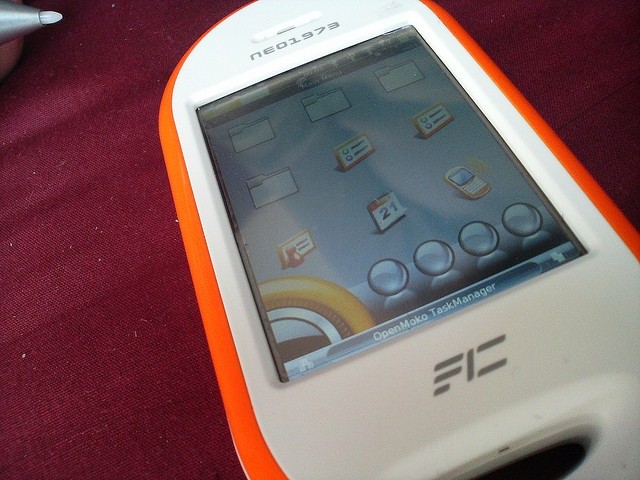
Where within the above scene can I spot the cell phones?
[157,0,640,480]
[445,166,492,201]
